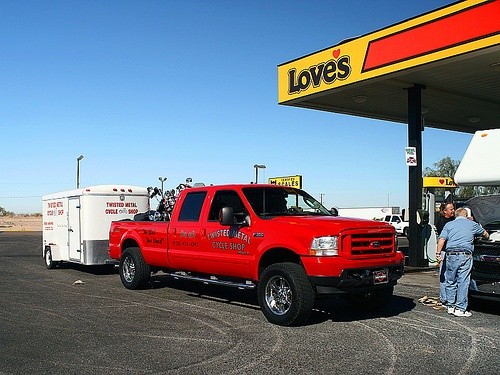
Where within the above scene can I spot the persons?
[433,202,489,317]
[158,200,171,222]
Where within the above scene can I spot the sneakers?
[454,309,472,317]
[447,306,456,315]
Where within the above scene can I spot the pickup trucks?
[373,214,411,236]
[108,182,406,326]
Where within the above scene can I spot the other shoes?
[442,303,448,309]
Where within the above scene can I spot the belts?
[447,252,471,255]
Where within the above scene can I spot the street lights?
[159,177,167,198]
[76,155,84,189]
[253,164,266,184]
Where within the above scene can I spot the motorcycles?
[132,183,193,220]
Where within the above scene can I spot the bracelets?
[436,252,441,256]
